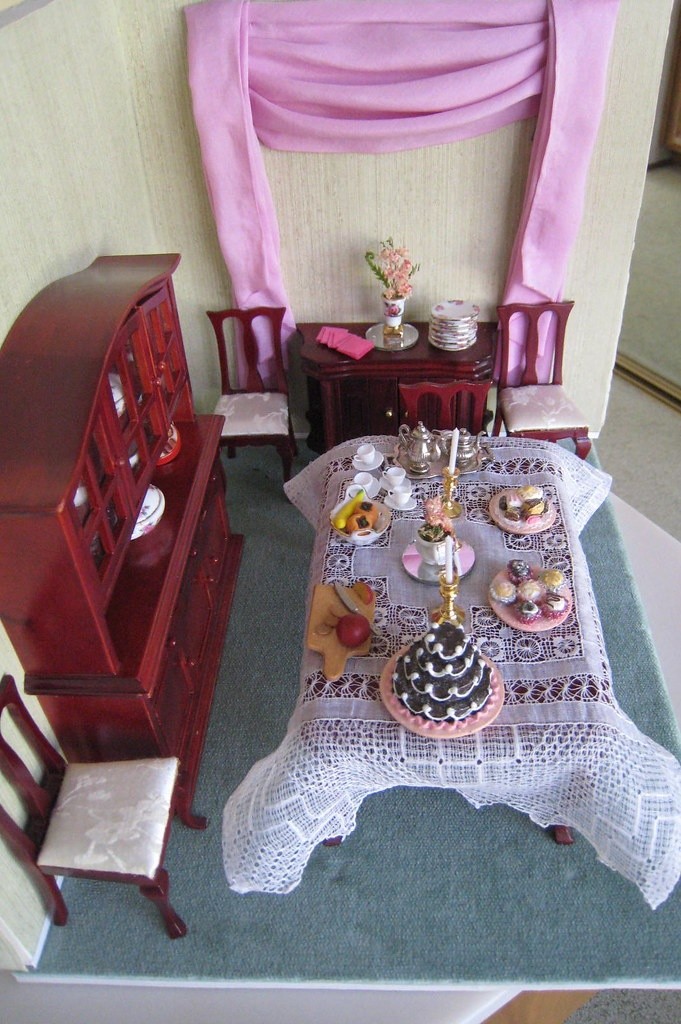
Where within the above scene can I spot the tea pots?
[399,421,441,474]
[441,428,487,468]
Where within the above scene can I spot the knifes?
[334,581,381,635]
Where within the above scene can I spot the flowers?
[364,236,421,300]
[417,496,455,542]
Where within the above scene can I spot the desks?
[221,436,681,912]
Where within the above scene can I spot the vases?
[381,293,406,338]
[415,537,455,566]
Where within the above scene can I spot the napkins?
[316,324,375,360]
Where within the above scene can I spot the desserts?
[498,484,549,522]
[490,560,569,624]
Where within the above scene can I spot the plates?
[379,477,411,491]
[383,495,417,511]
[349,478,380,499]
[489,490,556,534]
[352,451,383,471]
[428,300,480,351]
[379,646,505,739]
[487,567,573,632]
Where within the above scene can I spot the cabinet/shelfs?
[0,253,246,829]
[296,320,504,454]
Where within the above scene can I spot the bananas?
[333,489,364,527]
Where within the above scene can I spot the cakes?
[392,622,493,721]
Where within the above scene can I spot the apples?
[353,582,373,603]
[336,613,370,647]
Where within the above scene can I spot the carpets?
[12,435,681,985]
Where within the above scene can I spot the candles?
[445,535,454,585]
[448,427,459,475]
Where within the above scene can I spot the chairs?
[492,300,591,463]
[0,674,208,939]
[204,306,293,483]
[397,382,493,434]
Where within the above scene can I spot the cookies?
[316,608,347,635]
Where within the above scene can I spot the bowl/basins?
[131,483,165,540]
[329,484,391,546]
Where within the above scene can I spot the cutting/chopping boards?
[307,584,376,681]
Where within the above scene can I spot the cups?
[388,485,412,506]
[353,472,373,492]
[354,444,376,465]
[382,466,406,486]
[414,521,445,566]
